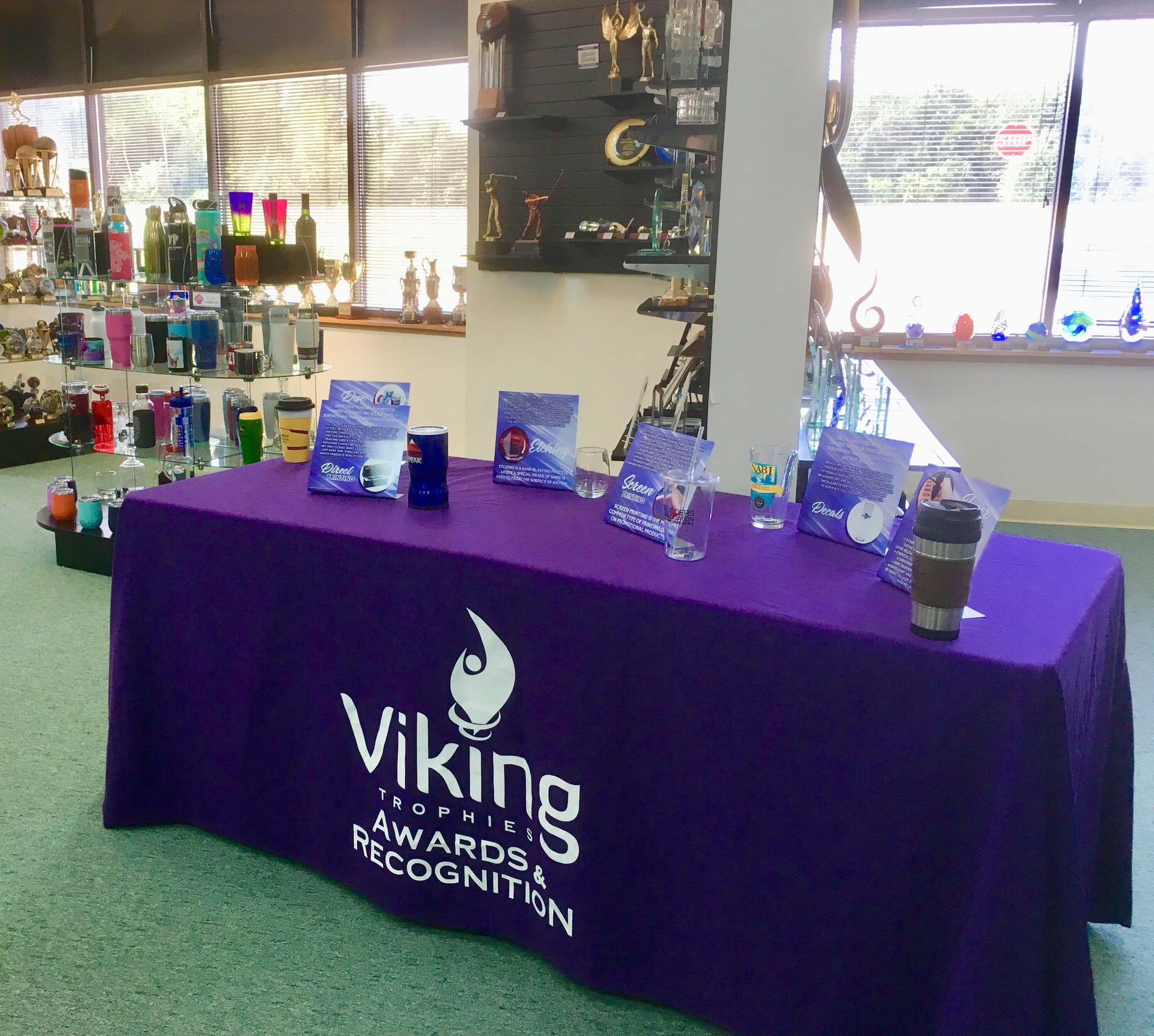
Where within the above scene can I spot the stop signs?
[994,122,1035,161]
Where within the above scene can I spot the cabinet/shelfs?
[35,266,332,578]
[462,0,722,271]
[612,0,732,464]
[0,193,101,468]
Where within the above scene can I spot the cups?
[660,469,721,560]
[130,334,155,367]
[133,410,156,449]
[234,349,273,376]
[909,500,982,640]
[205,248,229,284]
[166,337,203,372]
[573,447,609,498]
[79,338,105,367]
[407,425,450,510]
[46,472,123,532]
[228,342,254,371]
[275,397,312,463]
[111,402,134,431]
[750,445,798,529]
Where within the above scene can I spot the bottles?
[51,169,326,465]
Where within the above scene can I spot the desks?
[125,451,1134,1036]
[795,360,961,505]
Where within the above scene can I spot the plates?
[0,277,66,422]
